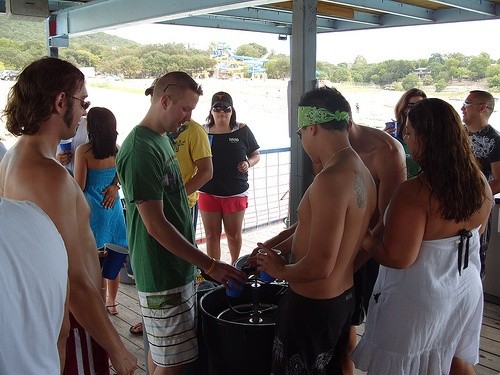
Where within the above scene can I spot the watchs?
[111,181,121,189]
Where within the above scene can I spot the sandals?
[129,322,143,336]
[106,305,119,315]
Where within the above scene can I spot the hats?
[210,91,233,108]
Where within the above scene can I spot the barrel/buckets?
[197,282,284,375]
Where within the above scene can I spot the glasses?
[212,105,231,112]
[163,83,202,93]
[462,101,481,108]
[296,126,306,140]
[72,96,91,110]
[406,103,416,108]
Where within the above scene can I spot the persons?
[348,119,406,318]
[197,92,259,266]
[115,71,243,375]
[384,87,426,181]
[0,56,138,375]
[353,98,494,375]
[245,87,377,375]
[461,90,500,283]
[144,75,213,247]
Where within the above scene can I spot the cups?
[102,243,129,280]
[208,135,213,146]
[60,142,71,152]
[385,122,397,138]
[258,249,281,282]
[226,277,243,298]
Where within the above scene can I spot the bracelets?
[245,159,250,167]
[204,258,216,275]
